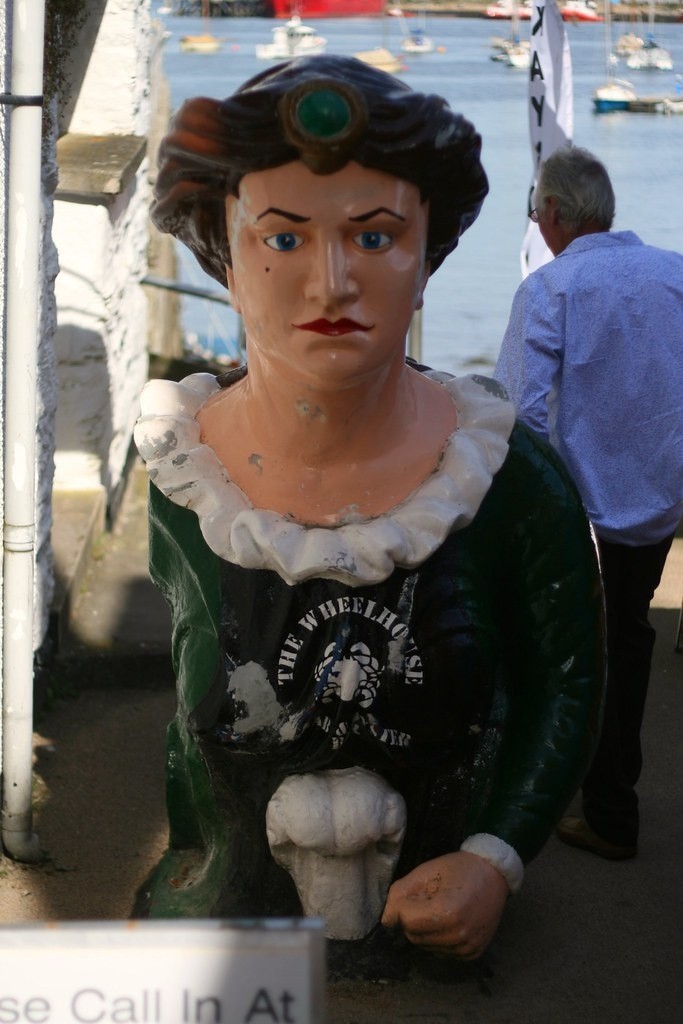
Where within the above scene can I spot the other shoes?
[557,816,639,861]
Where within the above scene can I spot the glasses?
[527,205,543,223]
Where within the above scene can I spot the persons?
[35,54,604,953]
[504,146,683,860]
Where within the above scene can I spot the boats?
[402,29,433,53]
[483,0,532,20]
[559,1,603,22]
[255,15,327,61]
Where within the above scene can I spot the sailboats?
[490,0,532,68]
[592,0,683,115]
